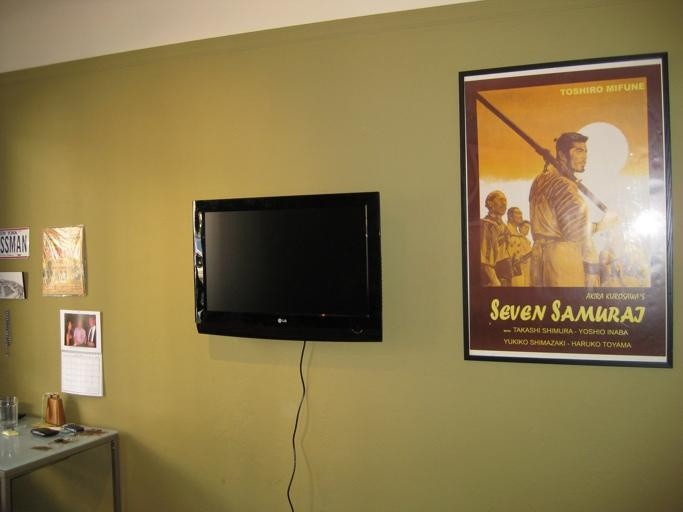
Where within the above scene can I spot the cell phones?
[63,424,83,433]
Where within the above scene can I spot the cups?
[0,397,19,428]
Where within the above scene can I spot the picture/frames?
[457,52,674,368]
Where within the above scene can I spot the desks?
[1,417,121,510]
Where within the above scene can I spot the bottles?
[41,393,66,427]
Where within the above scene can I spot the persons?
[66,317,96,347]
[480,133,620,288]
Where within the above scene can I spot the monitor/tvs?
[193,191,382,342]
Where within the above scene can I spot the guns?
[477,94,609,213]
